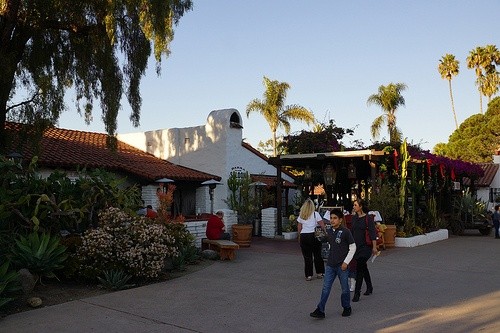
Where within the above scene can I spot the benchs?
[201,238,239,260]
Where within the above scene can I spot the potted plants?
[367,182,398,248]
[224,171,264,247]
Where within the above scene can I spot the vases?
[282,232,298,240]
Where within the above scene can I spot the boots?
[349,277,356,291]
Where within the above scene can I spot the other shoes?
[342,307,352,317]
[364,287,373,295]
[310,308,325,318]
[352,293,360,302]
[306,275,313,280]
[316,274,325,278]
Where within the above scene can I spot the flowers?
[282,215,296,232]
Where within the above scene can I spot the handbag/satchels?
[313,211,328,242]
[365,214,381,246]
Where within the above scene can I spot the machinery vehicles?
[408,179,495,237]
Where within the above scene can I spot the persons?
[135,200,158,220]
[351,199,378,302]
[206,211,231,241]
[309,208,357,319]
[296,198,327,281]
[491,205,500,239]
